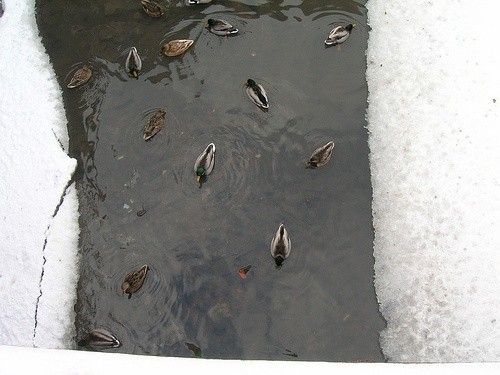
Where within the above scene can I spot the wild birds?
[189,0,212,4]
[142,110,167,140]
[203,18,239,36]
[125,47,142,78]
[270,224,292,267]
[160,39,194,57]
[82,329,120,348]
[243,78,270,108]
[67,64,93,89]
[307,141,334,168]
[193,143,216,182]
[120,264,148,294]
[139,0,165,17]
[324,23,356,45]
